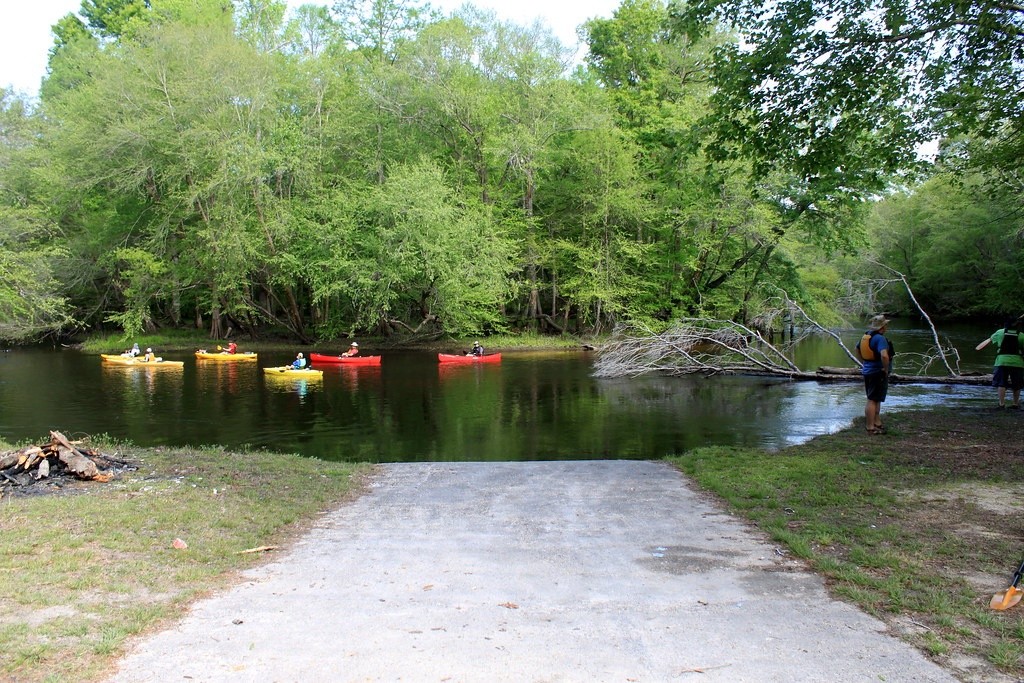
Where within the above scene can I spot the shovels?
[989,557,1024,611]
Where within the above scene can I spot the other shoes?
[1012,405,1020,408]
[998,403,1006,410]
[874,422,885,430]
[864,427,887,435]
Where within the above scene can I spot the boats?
[194,350,258,361]
[263,366,324,376]
[438,352,502,362]
[101,352,185,367]
[309,352,381,364]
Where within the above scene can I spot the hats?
[228,339,234,343]
[147,348,152,352]
[351,341,358,346]
[473,341,479,344]
[867,315,891,332]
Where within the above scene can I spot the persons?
[140,348,154,362]
[990,315,1024,411]
[473,341,484,354]
[286,352,306,370]
[221,340,237,354]
[121,343,140,358]
[341,341,359,357]
[856,315,895,435]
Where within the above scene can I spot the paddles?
[990,558,1024,611]
[974,312,1024,350]
[216,344,225,352]
